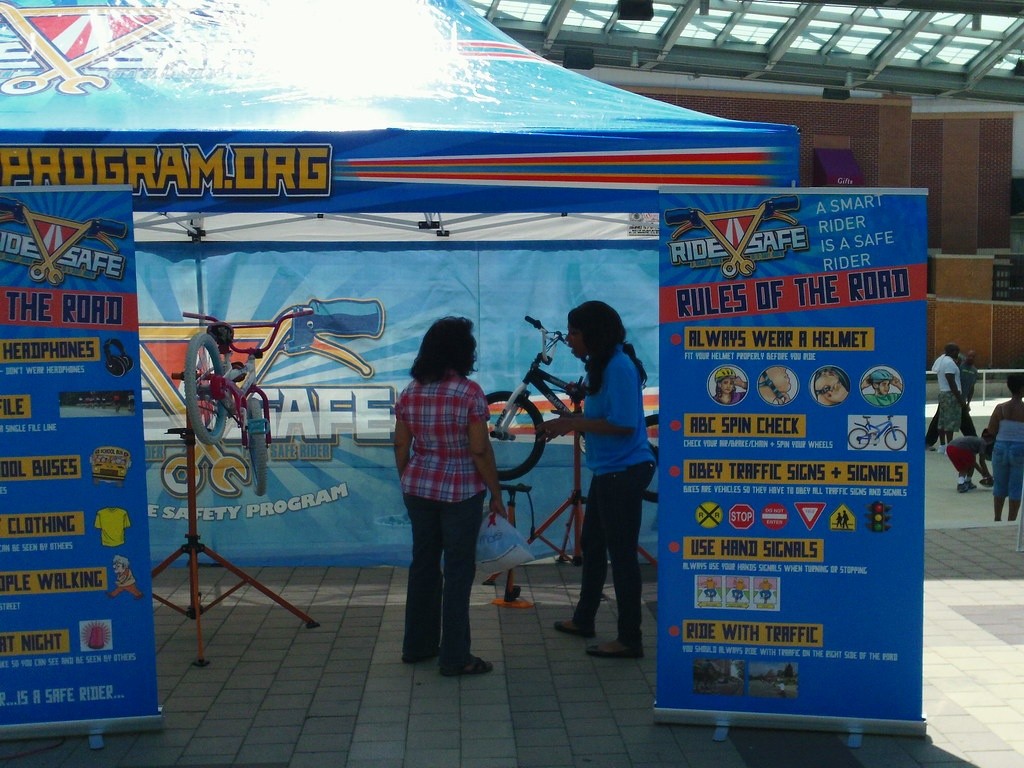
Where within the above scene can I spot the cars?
[848,414,908,451]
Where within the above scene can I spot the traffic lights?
[864,501,892,534]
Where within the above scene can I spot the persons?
[535,301,656,657]
[777,681,784,693]
[936,343,963,455]
[947,428,993,492]
[925,350,978,451]
[988,373,1024,521]
[393,317,507,675]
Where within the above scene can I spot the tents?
[0,0,800,567]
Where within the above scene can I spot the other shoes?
[963,480,977,489]
[957,483,969,492]
[402,646,441,663]
[586,645,643,658]
[555,622,595,636]
[937,445,946,454]
[925,444,935,451]
[440,657,492,676]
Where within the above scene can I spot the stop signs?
[761,505,790,530]
[729,504,755,530]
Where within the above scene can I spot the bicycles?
[174,302,317,496]
[471,314,663,504]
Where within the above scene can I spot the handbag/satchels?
[470,510,536,586]
